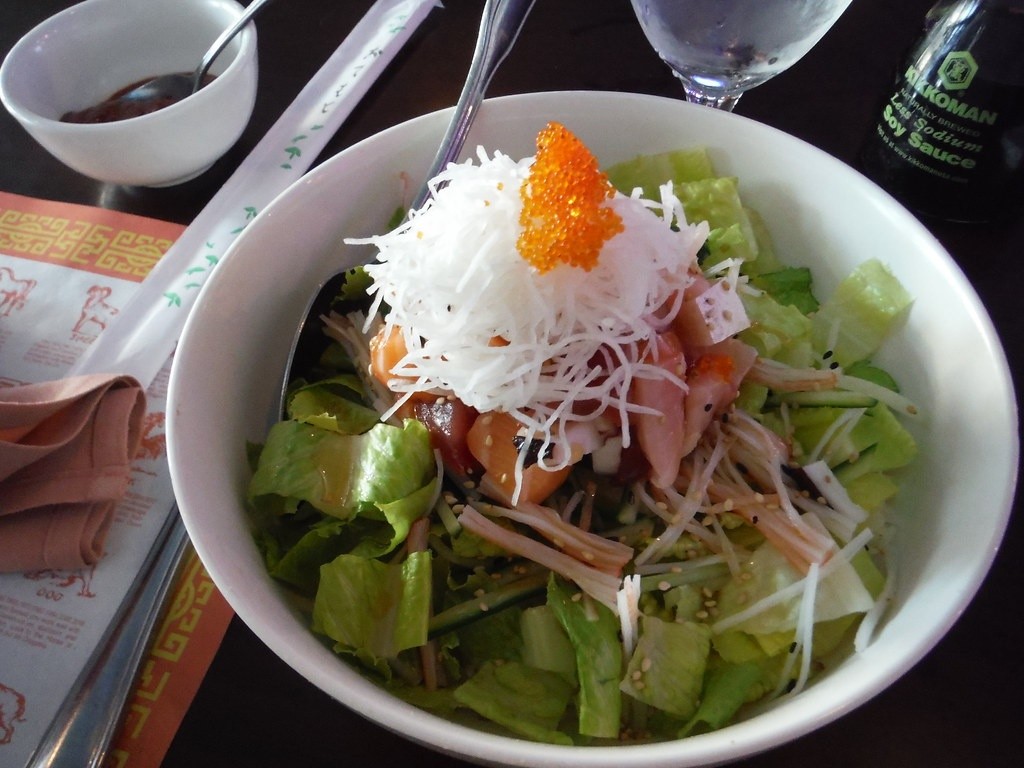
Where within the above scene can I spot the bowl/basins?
[0,0,258,188]
[166,90,1017,768]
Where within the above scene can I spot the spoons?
[279,2,538,425]
[118,0,270,98]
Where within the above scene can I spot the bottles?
[846,0,1024,224]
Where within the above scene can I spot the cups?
[628,0,855,112]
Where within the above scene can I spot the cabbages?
[239,146,915,745]
